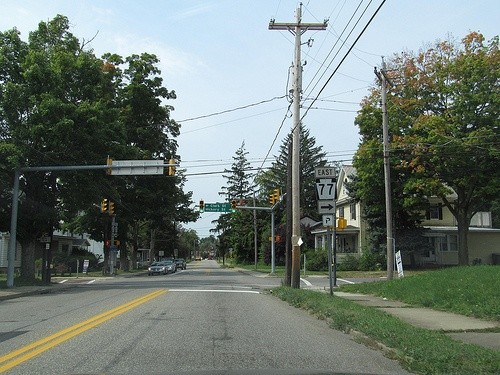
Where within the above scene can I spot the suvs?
[175,258,186,270]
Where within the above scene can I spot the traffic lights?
[101,199,108,213]
[231,200,237,213]
[109,201,114,216]
[199,201,204,213]
[106,240,110,246]
[274,188,280,201]
[270,194,275,205]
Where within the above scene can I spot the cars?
[148,261,176,275]
